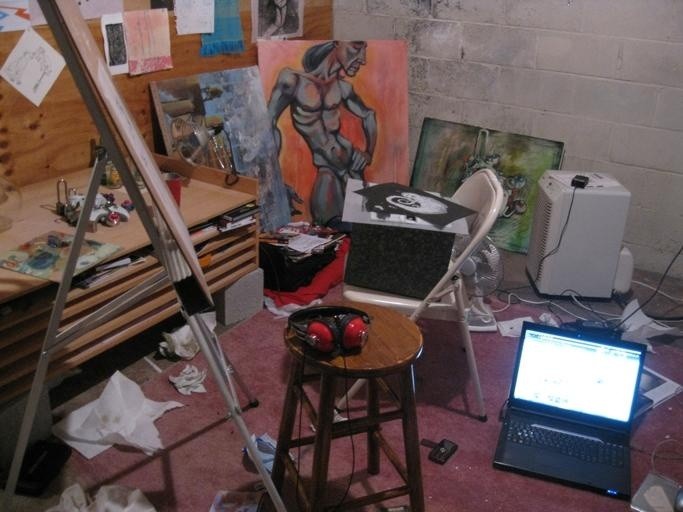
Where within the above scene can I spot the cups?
[162,171,180,209]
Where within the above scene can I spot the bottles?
[93,138,145,188]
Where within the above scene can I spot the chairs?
[330,167,506,423]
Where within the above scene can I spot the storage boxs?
[339,177,453,301]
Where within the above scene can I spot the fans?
[458,233,503,331]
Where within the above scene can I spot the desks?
[0,146,263,458]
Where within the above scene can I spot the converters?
[571,175,589,188]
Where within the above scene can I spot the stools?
[271,300,426,511]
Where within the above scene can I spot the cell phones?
[429,439,457,465]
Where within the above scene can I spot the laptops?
[493,321,647,500]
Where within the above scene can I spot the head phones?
[288,306,369,360]
